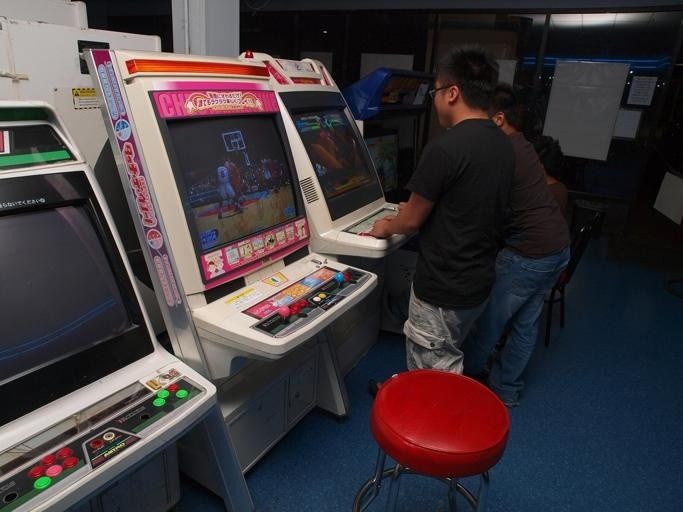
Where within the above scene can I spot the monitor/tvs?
[0,171,154,428]
[278,91,383,222]
[147,89,311,285]
[363,113,419,193]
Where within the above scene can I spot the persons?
[217,161,243,219]
[369,46,513,400]
[465,83,572,406]
[529,134,567,221]
[224,157,242,207]
[267,154,282,194]
[319,114,335,136]
[261,156,273,195]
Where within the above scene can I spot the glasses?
[429,83,461,98]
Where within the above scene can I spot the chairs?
[540,224,589,349]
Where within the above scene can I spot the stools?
[351,368,510,511]
